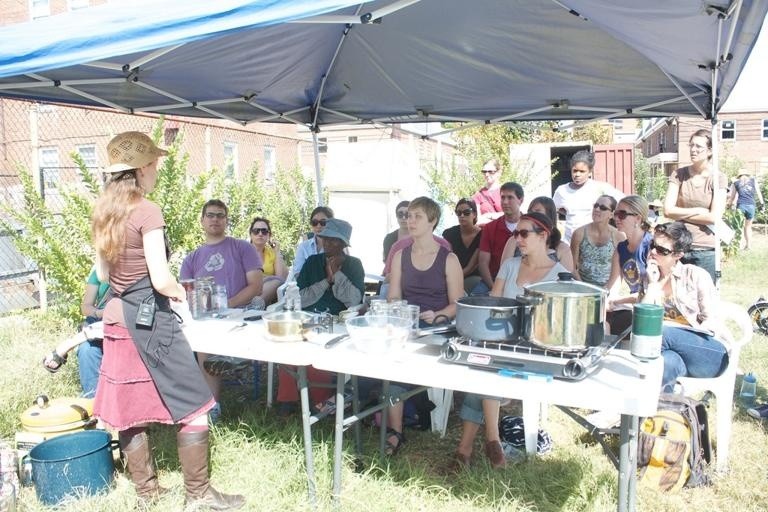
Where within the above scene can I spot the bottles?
[631,302,663,359]
[284,279,302,314]
[739,370,757,406]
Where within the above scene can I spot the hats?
[316,218,352,248]
[103,130,170,176]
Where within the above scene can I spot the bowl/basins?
[344,317,412,354]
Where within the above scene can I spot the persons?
[725,167,766,250]
[90,131,246,511]
[42,270,110,399]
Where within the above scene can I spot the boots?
[118,425,176,510]
[176,428,245,512]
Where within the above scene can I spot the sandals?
[313,393,352,417]
[385,426,405,457]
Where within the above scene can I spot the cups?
[370,297,421,331]
[179,277,230,319]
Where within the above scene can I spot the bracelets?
[93,308,98,321]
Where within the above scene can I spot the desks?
[169,300,362,502]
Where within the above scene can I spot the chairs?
[216,296,265,399]
[266,282,366,411]
[378,283,467,436]
[638,300,752,473]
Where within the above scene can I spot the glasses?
[251,228,269,236]
[310,218,327,227]
[613,209,638,220]
[481,170,499,175]
[649,240,676,257]
[204,211,226,219]
[512,228,543,239]
[396,211,408,219]
[594,203,611,212]
[454,207,473,217]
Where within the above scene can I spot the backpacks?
[636,379,714,491]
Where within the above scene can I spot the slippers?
[42,345,71,373]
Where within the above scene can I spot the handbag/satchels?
[495,412,553,459]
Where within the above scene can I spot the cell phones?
[244,315,262,321]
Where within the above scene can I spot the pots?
[452,294,524,341]
[17,395,119,509]
[519,270,609,353]
[262,311,306,343]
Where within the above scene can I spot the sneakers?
[209,403,221,423]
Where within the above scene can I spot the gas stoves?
[444,335,611,379]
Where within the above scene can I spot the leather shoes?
[436,450,471,477]
[485,439,508,472]
